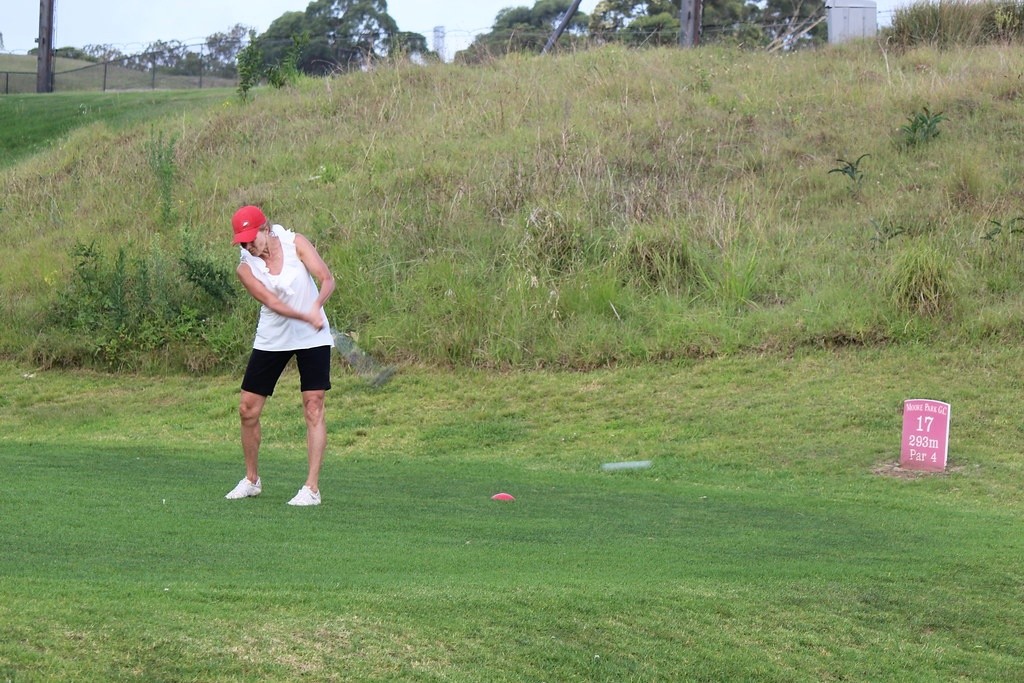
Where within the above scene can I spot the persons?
[226,206,336,506]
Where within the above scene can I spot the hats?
[232,206,266,246]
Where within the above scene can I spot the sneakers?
[225,476,262,499]
[287,485,321,506]
